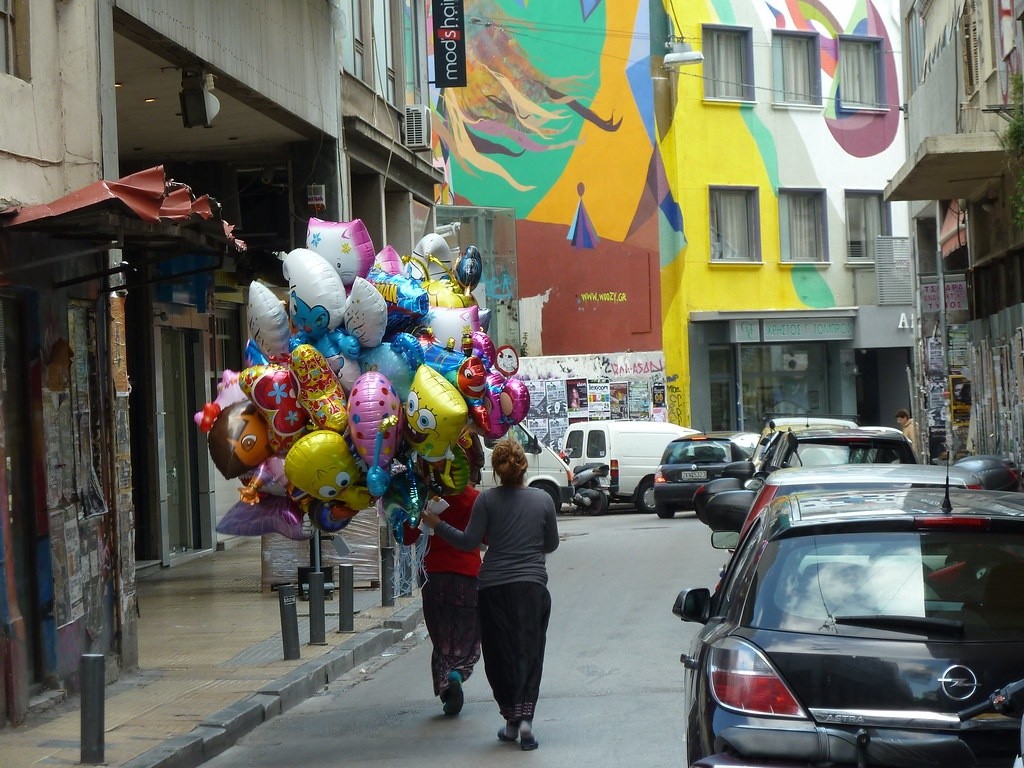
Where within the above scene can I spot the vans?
[563,419,730,513]
[470,418,576,514]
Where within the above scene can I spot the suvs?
[669,488,1024,768]
[691,414,986,597]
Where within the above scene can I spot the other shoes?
[443,670,464,715]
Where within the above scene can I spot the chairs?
[801,562,873,603]
[979,564,1024,613]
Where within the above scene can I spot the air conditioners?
[404,104,432,150]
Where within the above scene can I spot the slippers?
[521,736,538,750]
[498,728,514,742]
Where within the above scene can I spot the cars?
[654,429,762,519]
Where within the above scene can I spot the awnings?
[0,161,249,293]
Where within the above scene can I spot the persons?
[895,409,920,462]
[421,438,559,750]
[569,387,581,407]
[392,483,487,716]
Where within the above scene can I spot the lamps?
[663,36,704,67]
[179,69,221,127]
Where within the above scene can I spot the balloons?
[193,218,530,547]
